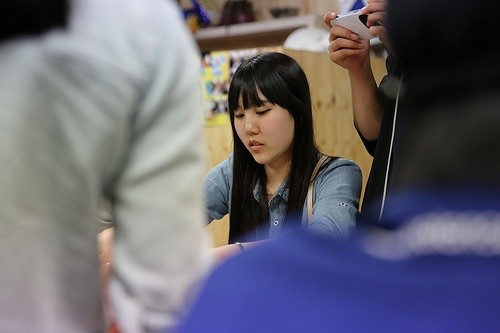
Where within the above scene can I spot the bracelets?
[234,241,248,255]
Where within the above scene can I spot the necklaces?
[264,187,280,197]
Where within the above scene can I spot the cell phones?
[330,7,384,40]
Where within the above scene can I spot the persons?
[322,0,403,232]
[169,0,500,332]
[0,0,209,333]
[96,50,363,313]
